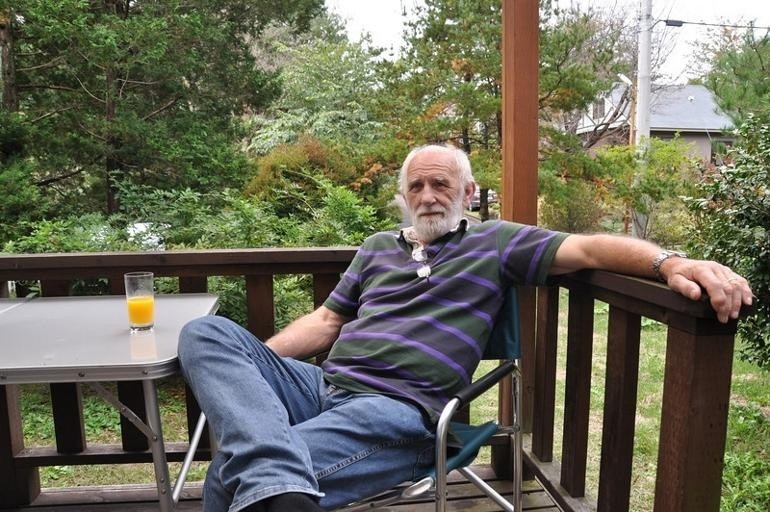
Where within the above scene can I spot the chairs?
[344,283,533,511]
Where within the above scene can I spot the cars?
[468,185,497,211]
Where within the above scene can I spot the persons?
[177,143,753,512]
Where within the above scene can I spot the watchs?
[651,249,688,285]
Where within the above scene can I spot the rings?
[726,277,737,282]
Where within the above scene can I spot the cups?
[124,271,155,332]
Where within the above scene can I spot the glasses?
[411,246,433,286]
